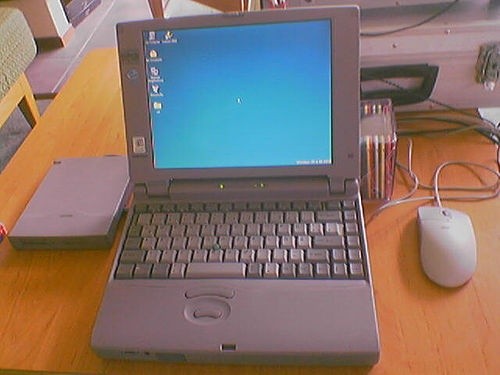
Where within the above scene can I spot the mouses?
[417,205,477,288]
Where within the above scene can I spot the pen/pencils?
[360,103,392,201]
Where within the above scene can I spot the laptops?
[90,4,380,365]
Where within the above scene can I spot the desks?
[1,45,500,375]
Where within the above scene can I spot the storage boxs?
[360,2,500,113]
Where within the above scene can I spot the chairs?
[2,5,42,137]
